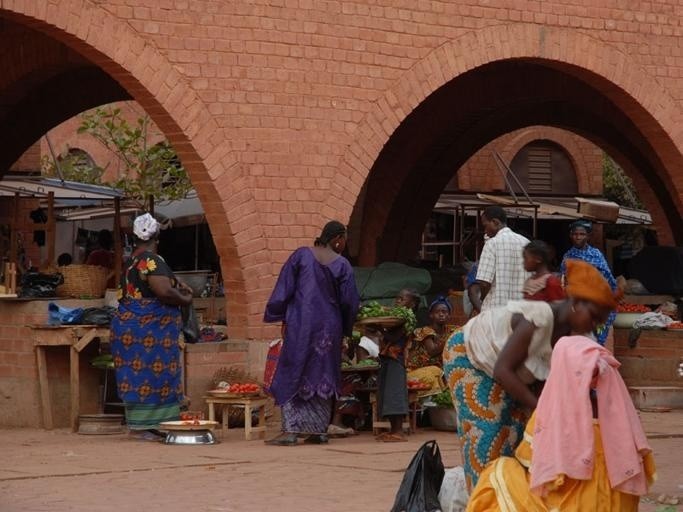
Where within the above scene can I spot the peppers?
[355,301,390,320]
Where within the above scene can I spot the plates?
[204,388,260,399]
[159,420,222,430]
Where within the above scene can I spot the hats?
[569,218,591,231]
[564,257,617,308]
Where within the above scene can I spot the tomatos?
[228,382,263,396]
[408,380,432,390]
[615,302,652,312]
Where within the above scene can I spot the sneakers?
[306,434,330,444]
[327,423,355,436]
[264,433,296,447]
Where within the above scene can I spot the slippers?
[131,429,164,443]
[373,429,406,443]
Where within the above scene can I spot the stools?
[203,395,269,442]
[369,392,417,436]
[0,262,18,298]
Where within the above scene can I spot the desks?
[24,324,111,433]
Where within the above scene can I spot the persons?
[522,240,567,301]
[462,334,657,512]
[442,258,617,499]
[560,221,616,349]
[475,206,536,313]
[58,229,125,298]
[110,212,193,441]
[468,260,490,318]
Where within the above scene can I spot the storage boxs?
[575,196,619,224]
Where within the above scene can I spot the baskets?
[211,366,268,427]
[49,262,109,299]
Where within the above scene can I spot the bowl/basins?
[612,313,651,329]
[163,432,220,446]
[171,269,214,299]
